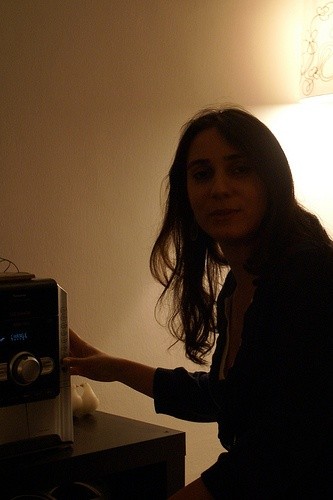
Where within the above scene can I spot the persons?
[58,105,332,500]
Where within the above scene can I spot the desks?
[2,407,188,499]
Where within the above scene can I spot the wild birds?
[71,382,99,419]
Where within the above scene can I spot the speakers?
[0,278,75,462]
